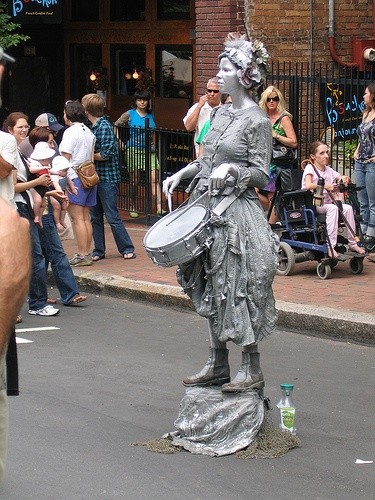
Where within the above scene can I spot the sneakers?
[69,253,93,266]
[28,305,60,316]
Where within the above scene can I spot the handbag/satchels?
[76,160,100,189]
[271,115,297,163]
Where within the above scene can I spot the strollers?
[266,168,370,279]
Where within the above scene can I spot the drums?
[143,204,215,268]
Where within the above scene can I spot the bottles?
[277,384,297,437]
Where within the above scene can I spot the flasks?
[315,177,324,206]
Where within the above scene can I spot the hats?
[35,113,64,131]
[50,156,73,171]
[29,141,56,160]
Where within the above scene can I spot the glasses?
[65,100,74,105]
[11,125,30,129]
[207,89,219,93]
[267,96,279,102]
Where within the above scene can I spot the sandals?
[46,299,57,303]
[92,254,101,260]
[124,253,136,259]
[69,294,87,305]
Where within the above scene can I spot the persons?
[182,78,233,159]
[113,90,167,217]
[301,141,365,257]
[0,93,135,321]
[162,33,280,393]
[0,196,33,498]
[353,84,375,252]
[259,85,297,229]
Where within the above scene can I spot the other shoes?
[129,210,139,217]
[157,209,166,215]
[357,234,375,252]
